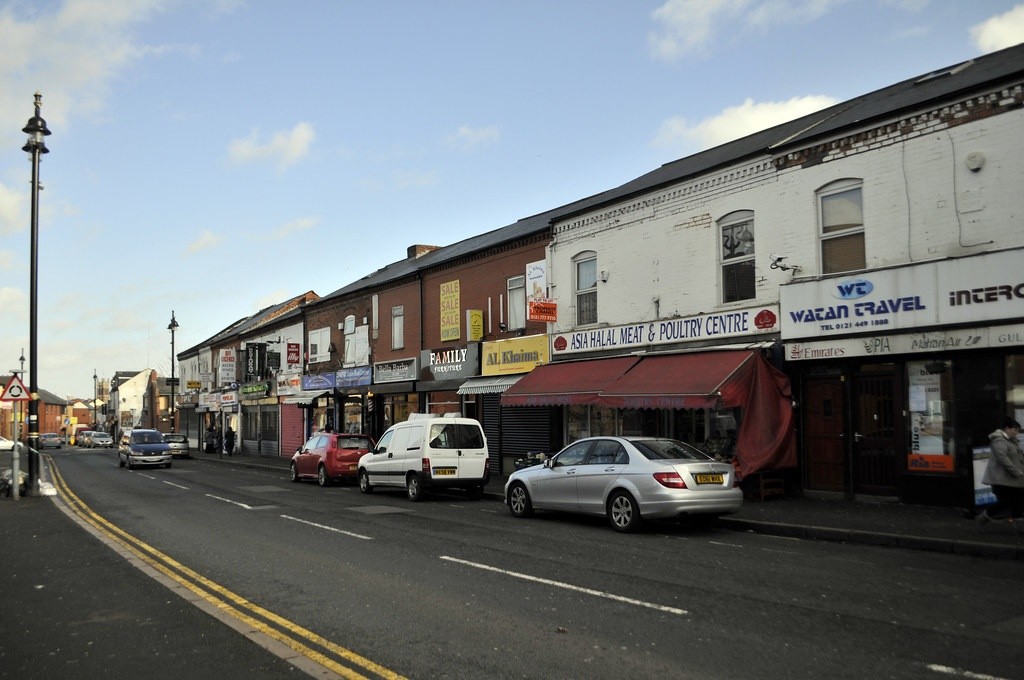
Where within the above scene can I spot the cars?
[503,434,746,535]
[161,433,190,458]
[117,426,173,470]
[0,435,24,453]
[38,428,114,449]
[288,432,378,487]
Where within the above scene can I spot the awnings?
[501,349,761,410]
[456,374,529,395]
[283,390,330,404]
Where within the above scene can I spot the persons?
[117,429,124,439]
[975,416,1024,526]
[205,426,235,457]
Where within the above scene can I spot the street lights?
[18,346,27,443]
[92,368,99,431]
[17,91,53,496]
[166,310,180,434]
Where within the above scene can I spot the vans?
[357,411,491,503]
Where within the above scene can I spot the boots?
[974,511,993,535]
